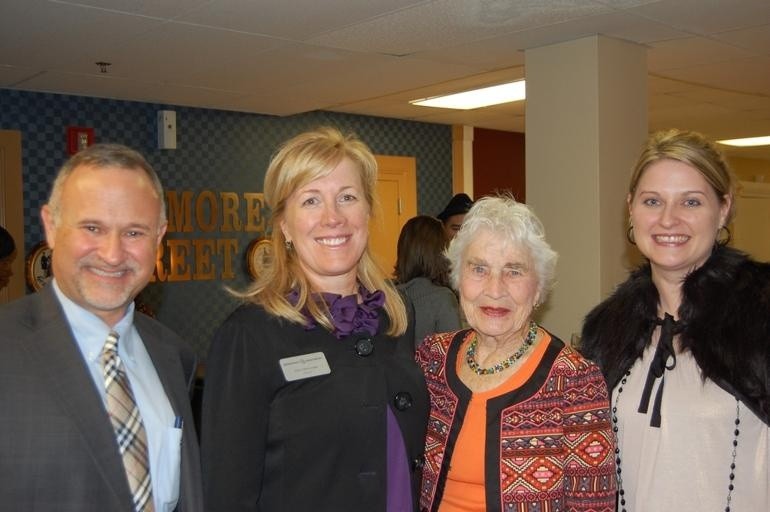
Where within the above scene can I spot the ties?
[102,331,156,511]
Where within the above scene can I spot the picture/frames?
[25,245,51,292]
[245,238,272,281]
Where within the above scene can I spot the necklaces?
[466,320,537,375]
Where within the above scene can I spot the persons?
[199,125,431,511]
[390,215,461,348]
[0,225,17,289]
[436,192,474,241]
[415,195,616,511]
[41,252,47,276]
[579,127,770,512]
[0,143,205,512]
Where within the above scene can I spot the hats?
[436,193,474,219]
